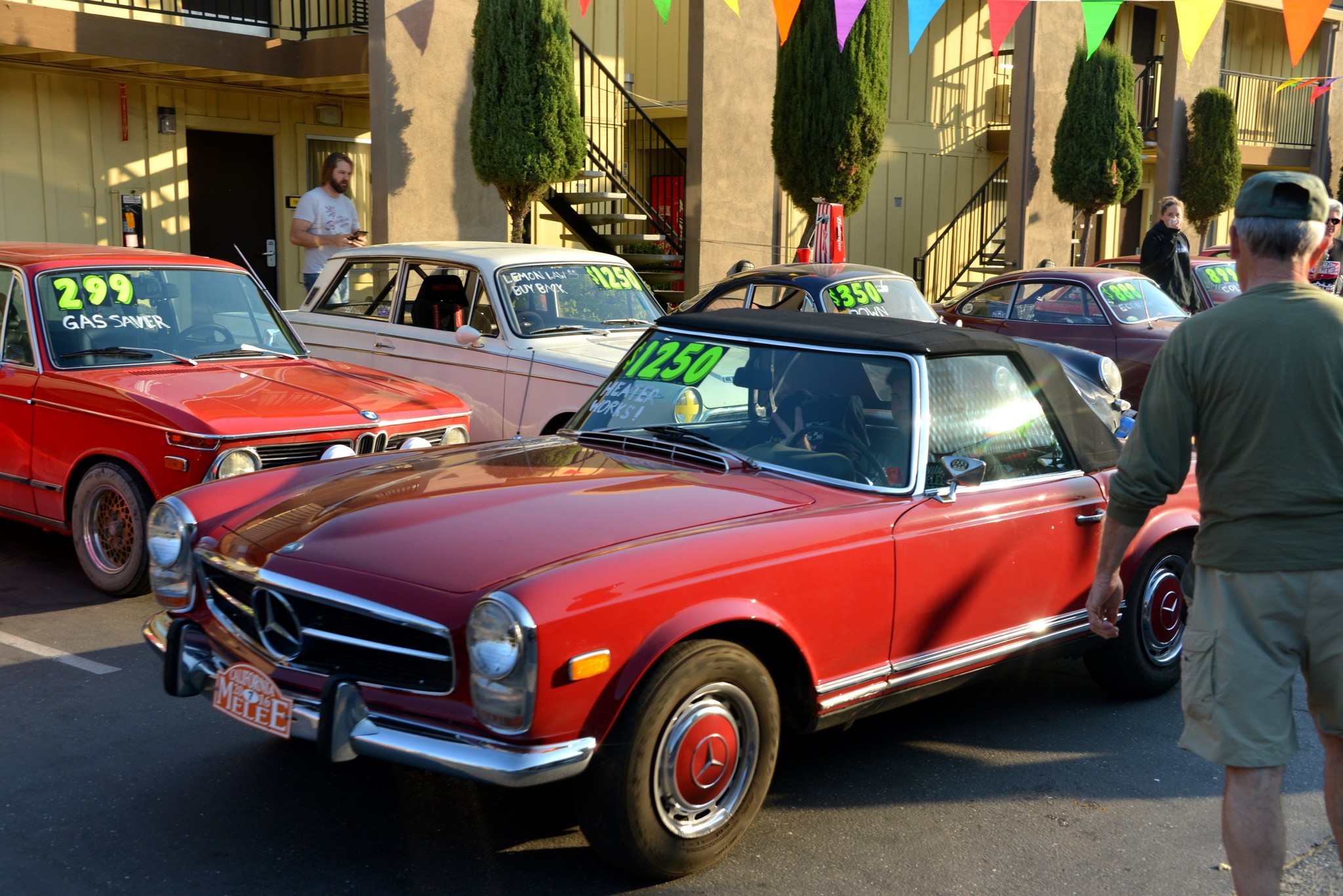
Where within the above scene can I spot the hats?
[1234,170,1330,222]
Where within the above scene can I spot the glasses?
[1326,218,1341,225]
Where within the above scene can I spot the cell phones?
[350,230,368,242]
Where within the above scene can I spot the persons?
[289,152,368,311]
[1086,173,1343,896]
[1141,195,1343,312]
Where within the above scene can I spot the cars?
[2,242,472,596]
[213,242,765,446]
[990,255,1245,314]
[1198,244,1232,259]
[142,308,1204,884]
[911,265,1198,418]
[658,263,1123,438]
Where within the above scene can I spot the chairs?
[411,273,493,335]
[759,383,874,463]
[0,298,181,365]
[962,300,1100,326]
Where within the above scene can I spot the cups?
[797,248,810,262]
